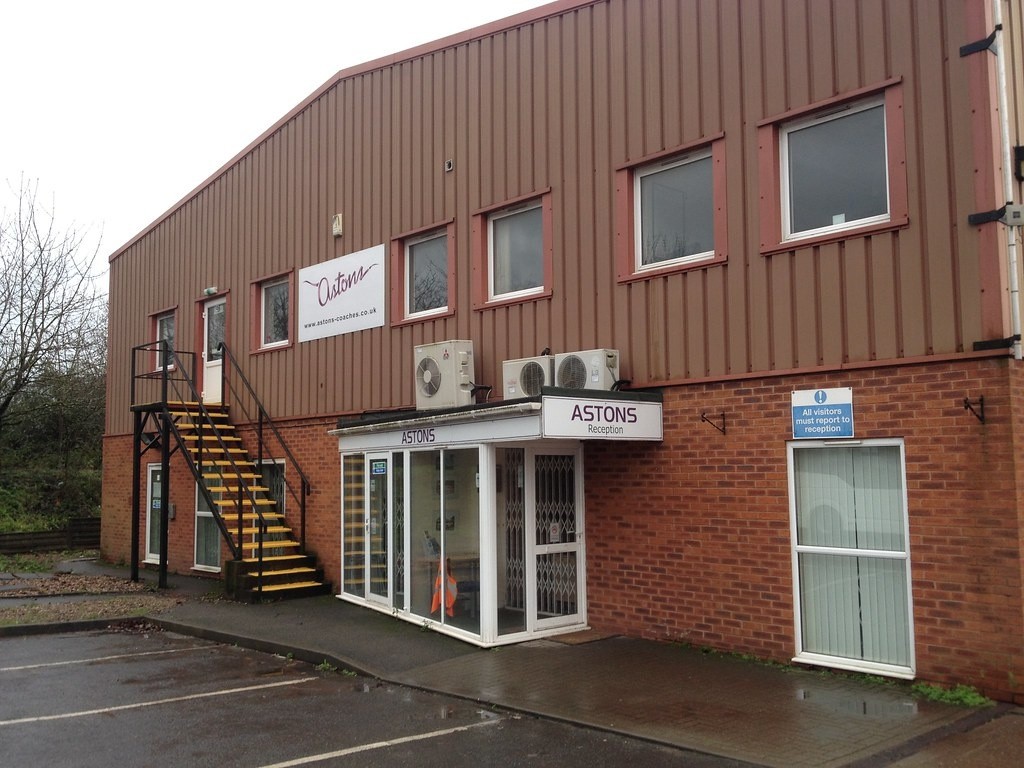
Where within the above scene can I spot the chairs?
[447,557,479,623]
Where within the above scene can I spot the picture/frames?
[431,452,458,474]
[431,475,459,500]
[432,510,460,536]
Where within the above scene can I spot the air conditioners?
[554,349,620,392]
[502,355,555,400]
[412,339,475,412]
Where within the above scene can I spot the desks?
[419,552,480,617]
